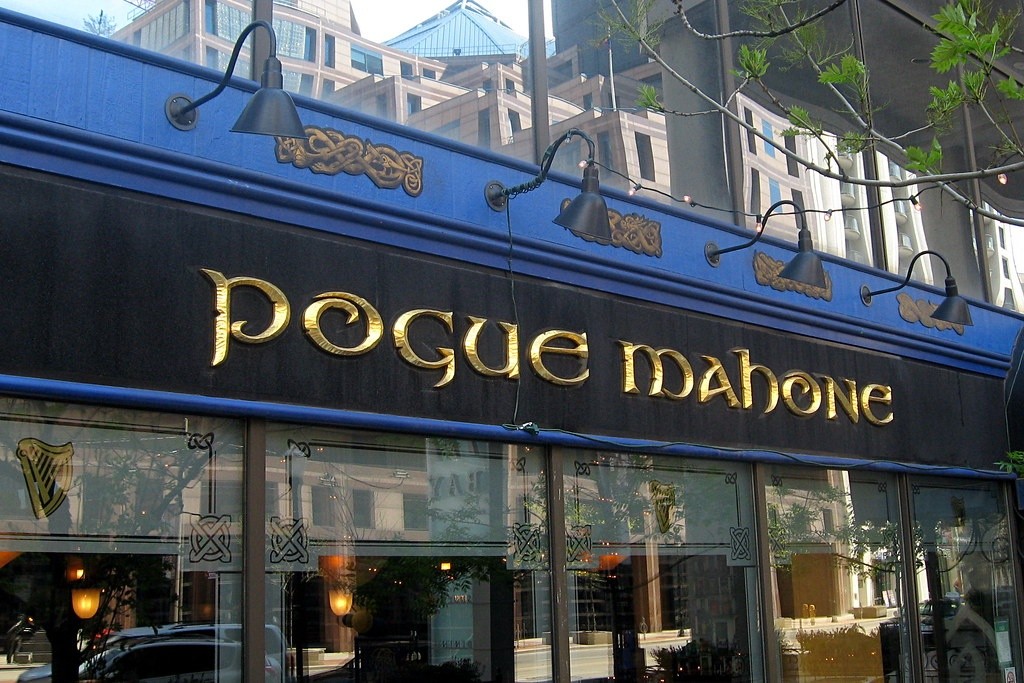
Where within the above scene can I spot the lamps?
[860,250,974,326]
[452,49,461,57]
[484,127,614,242]
[704,200,826,290]
[165,19,307,140]
[328,588,353,616]
[71,588,100,620]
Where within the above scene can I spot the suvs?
[78,637,279,683]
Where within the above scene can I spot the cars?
[875,591,970,634]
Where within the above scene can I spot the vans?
[15,619,295,683]
